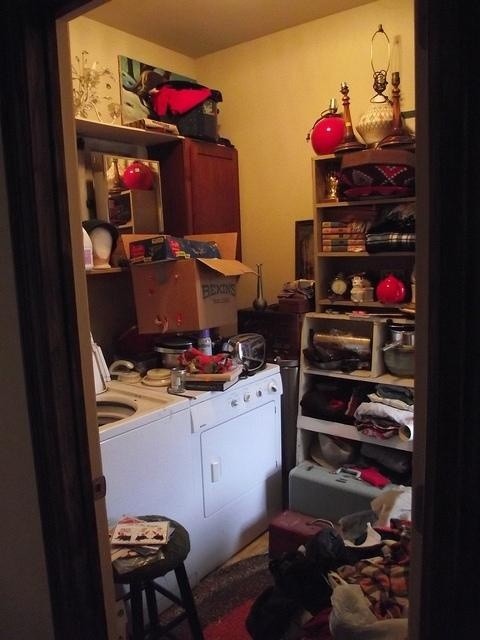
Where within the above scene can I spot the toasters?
[221,332,266,379]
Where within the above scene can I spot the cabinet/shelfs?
[74,117,181,275]
[107,189,159,234]
[148,137,243,281]
[310,142,417,312]
[294,310,415,467]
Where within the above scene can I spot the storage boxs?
[121,231,259,335]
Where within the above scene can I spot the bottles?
[199,330,213,356]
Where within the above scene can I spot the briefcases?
[268,509,338,563]
[288,460,397,526]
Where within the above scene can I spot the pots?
[383,325,415,351]
[153,334,198,369]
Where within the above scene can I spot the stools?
[108,515,203,640]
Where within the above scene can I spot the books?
[109,515,175,561]
[183,366,243,391]
[322,221,366,252]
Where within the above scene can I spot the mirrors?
[78,136,164,235]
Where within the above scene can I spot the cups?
[170,368,186,392]
